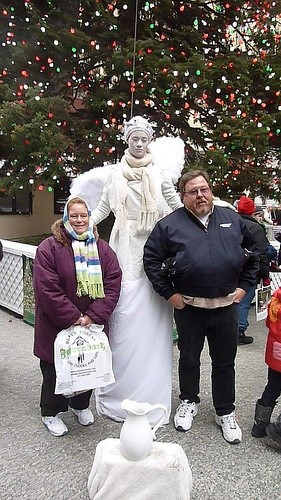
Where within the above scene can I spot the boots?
[251,399,275,438]
[265,413,281,442]
[237,329,254,345]
[270,260,281,272]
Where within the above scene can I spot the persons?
[232,195,281,345]
[143,171,260,444]
[66,115,186,427]
[34,198,123,437]
[250,287,281,451]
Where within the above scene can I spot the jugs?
[119,399,167,461]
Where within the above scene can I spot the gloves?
[263,277,270,286]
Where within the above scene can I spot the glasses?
[183,187,210,195]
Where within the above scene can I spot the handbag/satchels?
[54,323,116,395]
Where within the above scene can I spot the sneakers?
[41,415,68,437]
[174,399,198,431]
[215,404,242,444]
[72,408,94,426]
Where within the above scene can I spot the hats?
[237,196,256,215]
